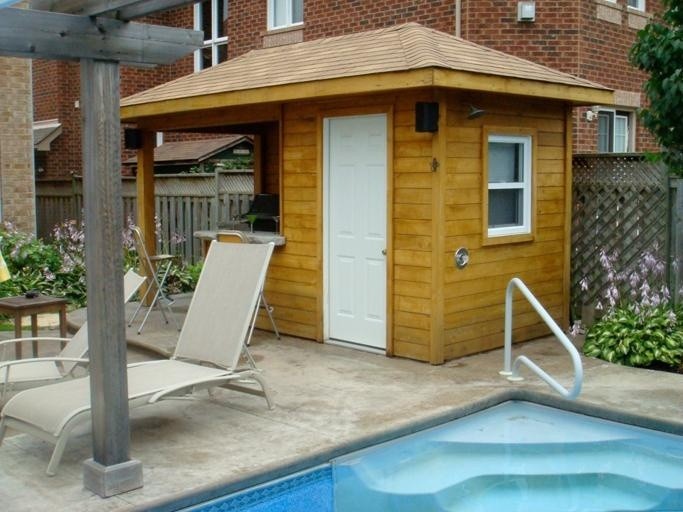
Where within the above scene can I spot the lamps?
[459,100,500,120]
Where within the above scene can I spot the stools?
[128,224,181,336]
[214,230,280,346]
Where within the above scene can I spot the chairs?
[1,239,274,475]
[2,267,146,404]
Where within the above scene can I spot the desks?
[2,296,69,360]
